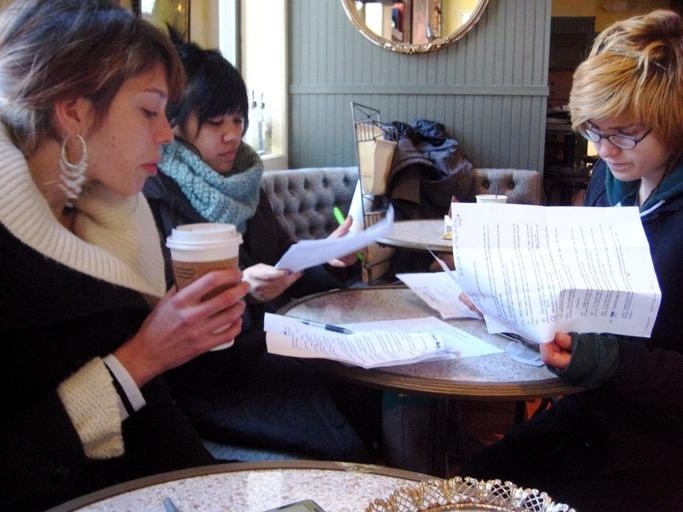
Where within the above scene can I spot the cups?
[475,194,508,203]
[165,222,245,353]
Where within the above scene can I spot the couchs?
[261,166,541,244]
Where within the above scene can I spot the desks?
[372,221,454,251]
[274,287,589,474]
[41,461,525,512]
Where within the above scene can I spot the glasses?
[583,120,656,149]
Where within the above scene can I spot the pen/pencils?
[286,315,353,335]
[332,206,365,262]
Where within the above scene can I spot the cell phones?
[266,499,324,512]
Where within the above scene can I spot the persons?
[141,25,438,472]
[448,10,683,512]
[0,1,251,512]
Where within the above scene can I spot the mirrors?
[341,0,491,55]
